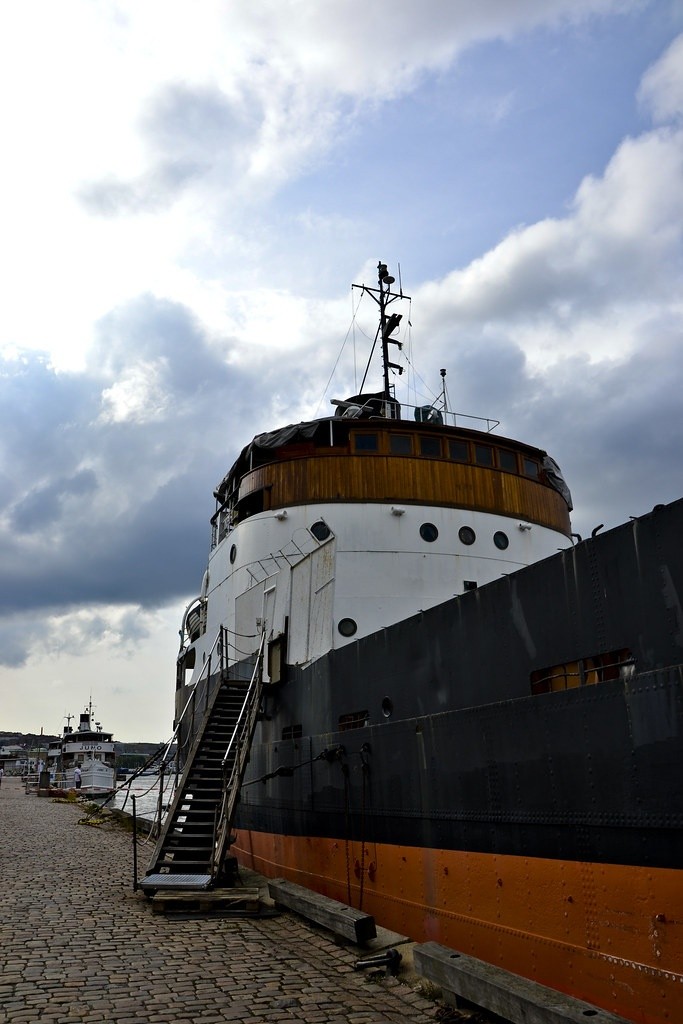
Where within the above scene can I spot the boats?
[136,260,682,1024]
[0,685,183,801]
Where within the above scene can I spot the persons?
[74,764,82,788]
